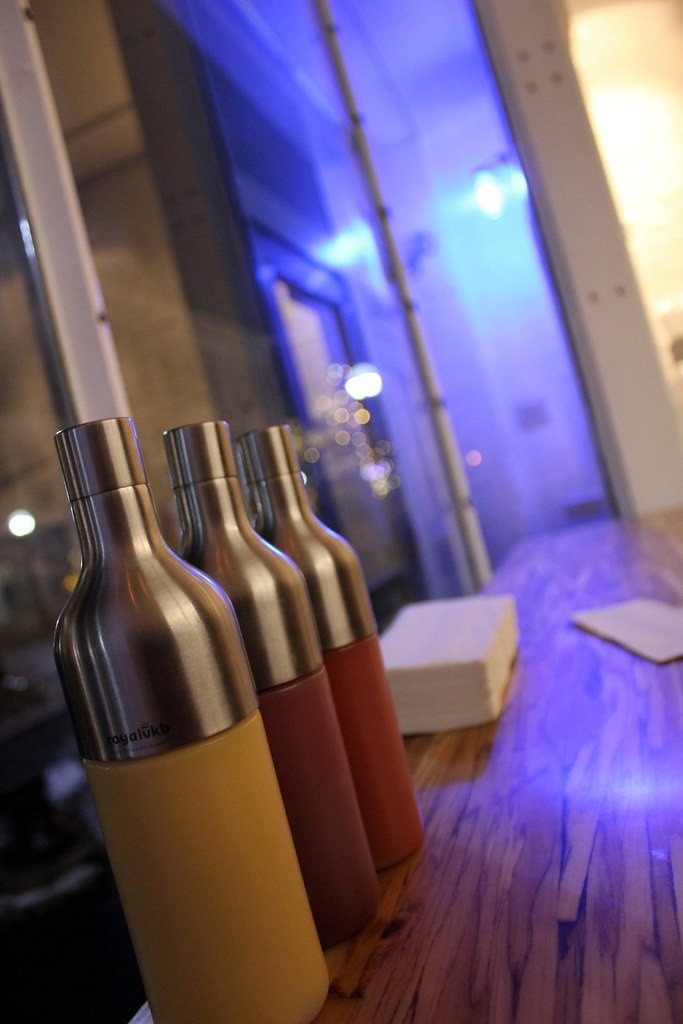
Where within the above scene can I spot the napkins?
[573,596,681,664]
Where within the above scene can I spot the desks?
[128,504,683,1024]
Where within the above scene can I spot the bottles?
[232,423,424,869]
[159,421,381,950]
[50,417,331,1024]
